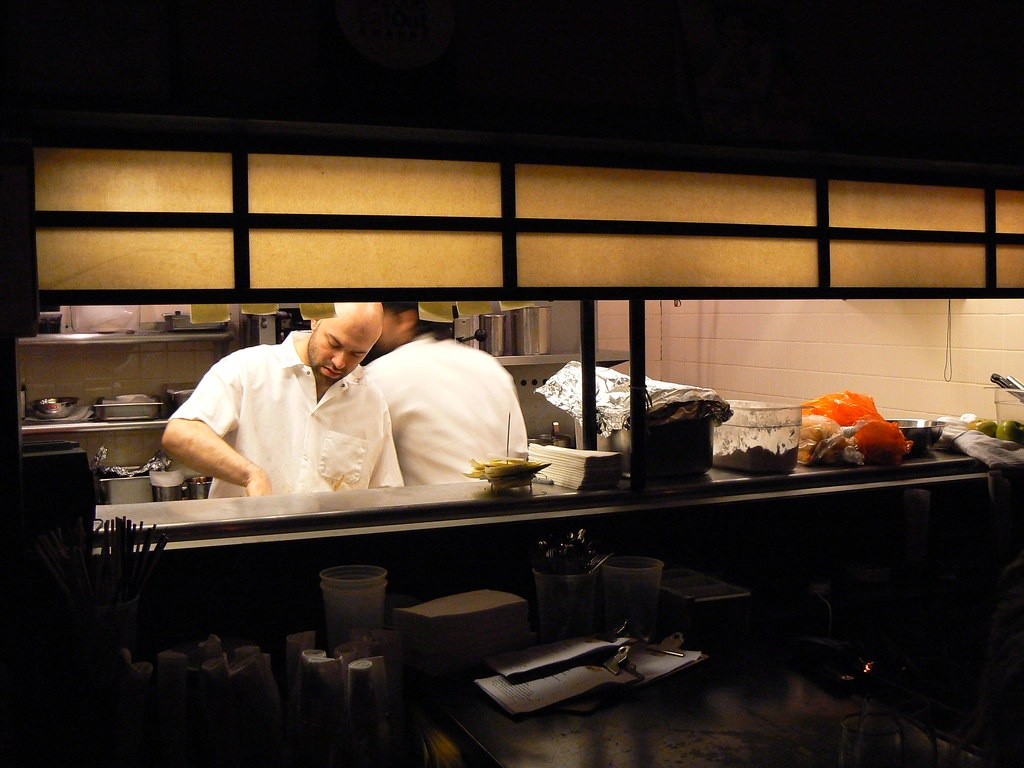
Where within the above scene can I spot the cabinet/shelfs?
[9,331,238,435]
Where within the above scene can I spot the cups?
[601,555,663,645]
[318,564,388,657]
[994,389,1024,426]
[532,567,598,643]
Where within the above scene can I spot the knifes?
[990,373,1024,403]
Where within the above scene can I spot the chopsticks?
[31,517,170,606]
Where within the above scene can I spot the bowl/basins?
[885,418,949,461]
[99,476,154,505]
[184,476,213,500]
[30,396,80,419]
[149,470,184,502]
[712,398,806,473]
[93,402,163,421]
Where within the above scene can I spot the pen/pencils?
[538,530,614,576]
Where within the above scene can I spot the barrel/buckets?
[478,314,506,356]
[510,307,551,354]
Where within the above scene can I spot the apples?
[966,417,1024,444]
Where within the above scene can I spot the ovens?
[239,307,311,348]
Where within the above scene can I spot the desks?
[403,644,987,768]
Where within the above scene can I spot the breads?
[796,416,855,467]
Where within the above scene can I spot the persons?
[163,303,404,499]
[362,303,528,486]
